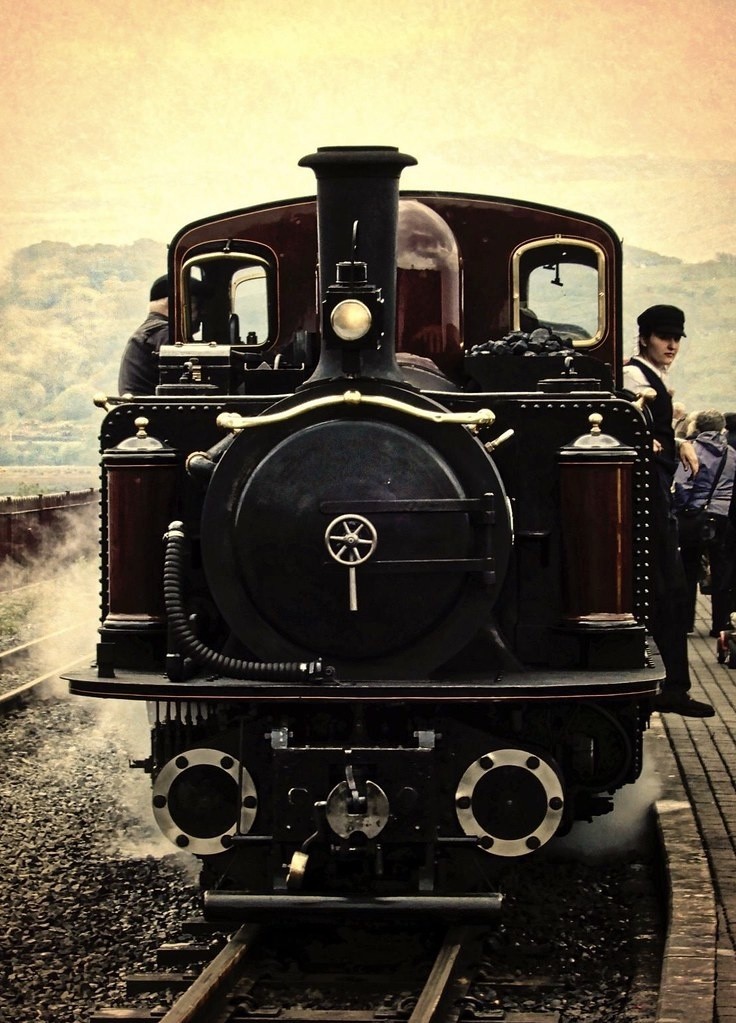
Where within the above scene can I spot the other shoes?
[687,624,694,633]
[656,690,715,718]
[709,628,723,638]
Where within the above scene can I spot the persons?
[622,305,716,718]
[672,403,736,670]
[118,275,170,395]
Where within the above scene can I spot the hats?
[637,305,687,338]
[150,273,211,301]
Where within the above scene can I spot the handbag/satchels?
[677,507,716,545]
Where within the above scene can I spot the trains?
[54,141,668,918]
